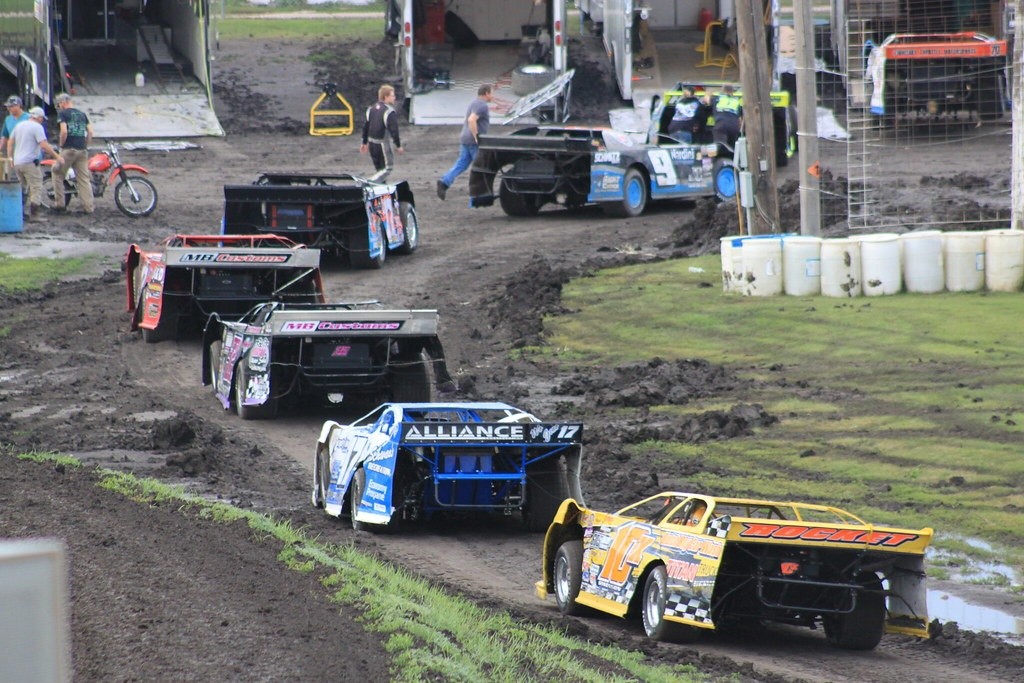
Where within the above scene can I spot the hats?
[721,85,738,92]
[4,95,23,107]
[54,94,71,109]
[28,106,49,121]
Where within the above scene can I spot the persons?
[361,85,403,185]
[0,95,31,151]
[7,106,65,221]
[668,87,706,145]
[52,93,96,213]
[436,84,494,201]
[957,82,983,128]
[703,85,743,150]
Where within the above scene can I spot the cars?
[864,28,1006,124]
[128,234,325,344]
[223,171,417,269]
[202,301,455,419]
[535,491,934,643]
[646,81,794,163]
[468,70,741,214]
[312,403,586,533]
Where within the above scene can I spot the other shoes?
[45,207,66,214]
[436,179,446,200]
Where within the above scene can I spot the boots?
[29,204,48,223]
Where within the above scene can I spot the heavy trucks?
[1,1,227,141]
[578,1,783,114]
[384,1,571,125]
[830,0,1011,127]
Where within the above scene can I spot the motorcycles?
[40,138,158,217]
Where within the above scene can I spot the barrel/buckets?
[0,179,24,233]
[721,228,1024,300]
[135,74,144,87]
